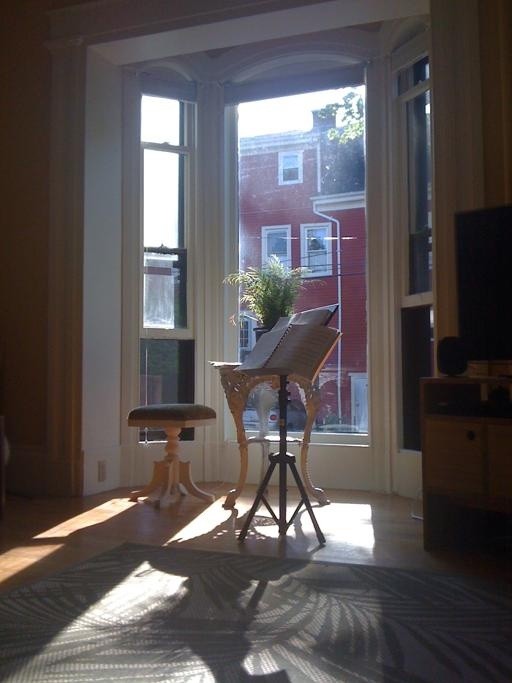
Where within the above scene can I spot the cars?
[243,396,307,430]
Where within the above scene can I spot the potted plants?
[220,253,328,344]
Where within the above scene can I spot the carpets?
[0,539,511,683]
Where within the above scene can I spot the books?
[234,323,343,386]
[272,310,332,326]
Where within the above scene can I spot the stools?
[126,402,218,508]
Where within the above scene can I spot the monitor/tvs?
[453,204,511,362]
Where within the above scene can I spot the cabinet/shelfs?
[418,359,512,551]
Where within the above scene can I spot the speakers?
[436,336,467,375]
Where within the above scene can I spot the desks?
[210,360,331,511]
